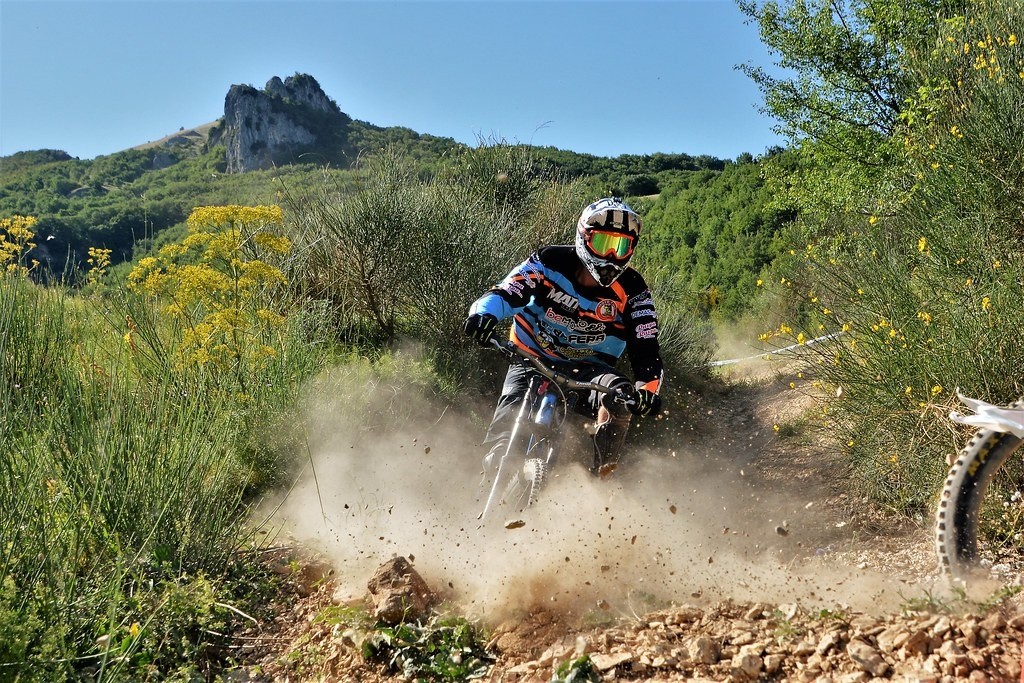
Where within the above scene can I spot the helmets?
[576,196,643,289]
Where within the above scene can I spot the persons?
[463,197,664,481]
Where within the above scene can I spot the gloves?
[633,381,661,425]
[460,313,498,346]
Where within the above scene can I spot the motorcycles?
[933,393,1024,592]
[460,318,639,517]
